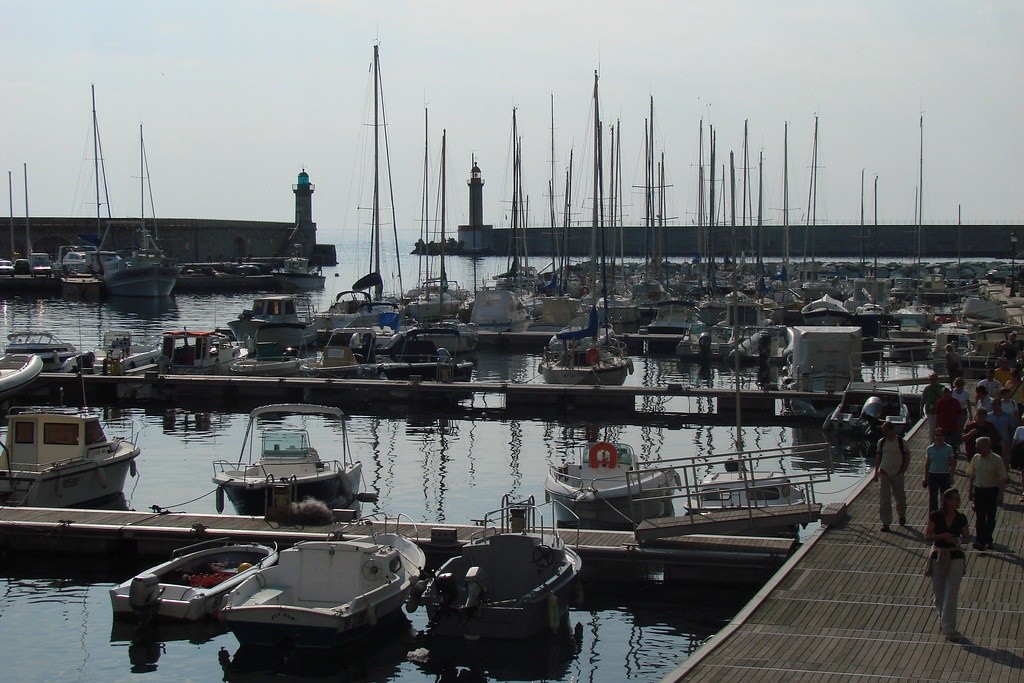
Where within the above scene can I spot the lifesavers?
[586,347,598,365]
[589,442,618,468]
[580,286,589,295]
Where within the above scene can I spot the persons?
[935,387,962,454]
[978,369,1001,397]
[925,489,970,640]
[974,386,992,411]
[920,374,945,444]
[922,428,956,514]
[1000,389,1019,417]
[986,397,1017,483]
[965,437,1007,550]
[873,422,910,532]
[994,333,1024,403]
[951,377,973,428]
[1011,426,1024,502]
[945,344,962,378]
[961,407,1001,463]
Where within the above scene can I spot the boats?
[419,492,586,638]
[0,309,150,507]
[0,352,44,406]
[217,511,427,636]
[211,402,378,520]
[108,536,280,622]
[540,440,683,531]
[820,379,912,451]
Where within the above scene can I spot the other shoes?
[944,630,961,639]
[986,541,994,549]
[973,543,986,551]
[899,514,906,526]
[880,524,889,531]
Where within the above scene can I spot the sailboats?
[1,20,1023,386]
[682,150,808,517]
